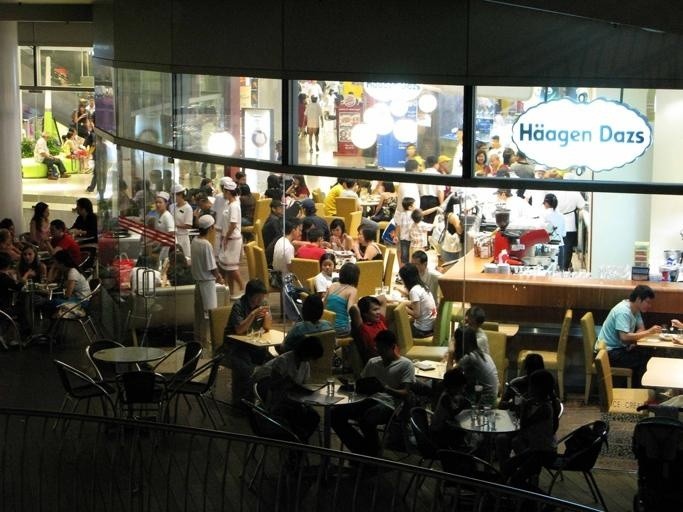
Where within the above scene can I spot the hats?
[439,155,452,163]
[199,214,215,229]
[270,200,283,207]
[544,194,557,203]
[493,188,512,194]
[220,177,237,191]
[302,198,315,209]
[156,191,170,201]
[171,185,186,193]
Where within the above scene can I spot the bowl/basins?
[34,285,47,290]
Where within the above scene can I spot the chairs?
[112,372,173,441]
[241,402,314,489]
[540,419,612,512]
[597,349,656,423]
[631,417,681,511]
[437,449,505,511]
[165,355,229,430]
[0,191,680,401]
[405,407,448,496]
[51,359,120,435]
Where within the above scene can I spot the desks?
[447,409,525,435]
[290,382,366,477]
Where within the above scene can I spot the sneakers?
[48,175,57,180]
[231,291,245,301]
[60,172,71,178]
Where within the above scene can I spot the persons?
[0,80,683,511]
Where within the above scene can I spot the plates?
[658,334,679,341]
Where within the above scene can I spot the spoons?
[670,320,674,332]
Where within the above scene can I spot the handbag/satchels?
[56,302,86,319]
[382,219,399,246]
[113,258,135,290]
[269,269,282,289]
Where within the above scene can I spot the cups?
[27,279,33,290]
[471,404,496,431]
[327,377,335,396]
[247,327,262,341]
[661,250,681,282]
[376,286,389,296]
[260,301,269,315]
